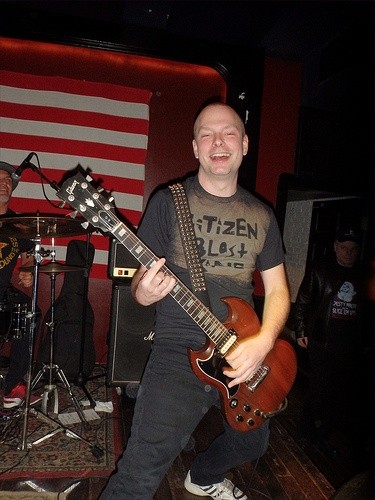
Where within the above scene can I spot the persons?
[0,161,50,409]
[292,220,375,487]
[95,102,291,500]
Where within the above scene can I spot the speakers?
[107,280,157,387]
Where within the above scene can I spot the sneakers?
[3,383,41,408]
[184,470,247,500]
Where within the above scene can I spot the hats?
[334,223,361,243]
[0,161,18,191]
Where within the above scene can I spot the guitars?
[55,166,298,433]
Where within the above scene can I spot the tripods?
[0,219,106,461]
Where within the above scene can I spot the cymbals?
[17,261,87,274]
[0,215,94,240]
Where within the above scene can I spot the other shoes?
[305,419,327,441]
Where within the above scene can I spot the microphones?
[11,152,34,180]
